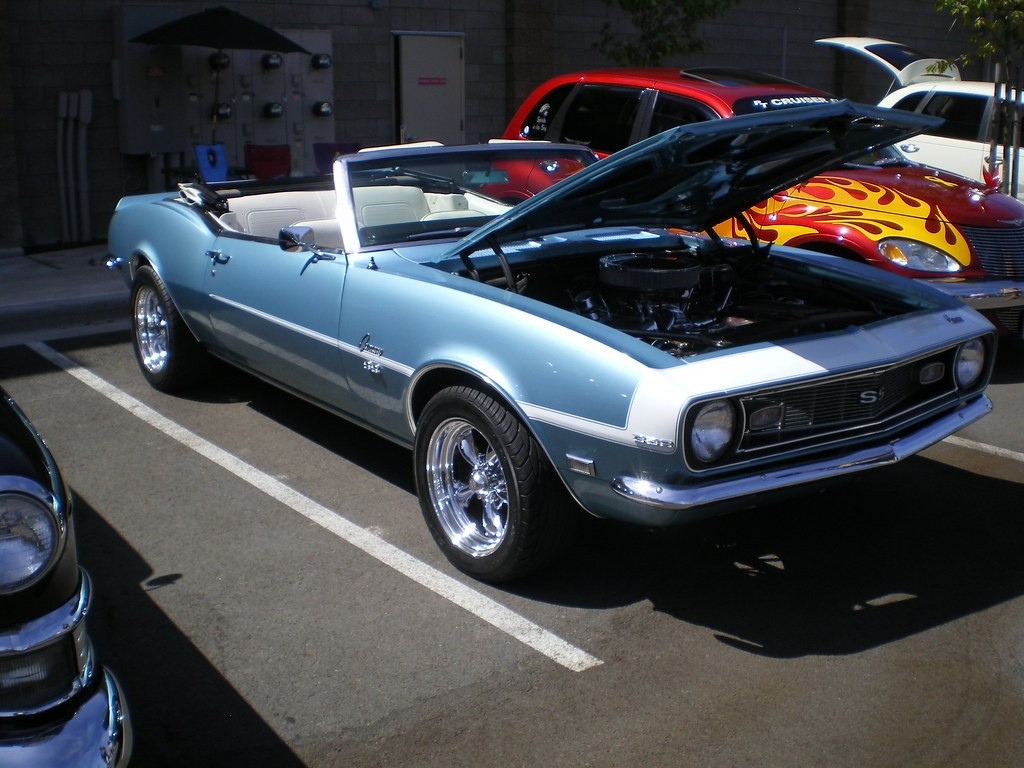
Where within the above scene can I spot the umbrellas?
[128,5,313,146]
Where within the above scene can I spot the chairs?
[420,209,488,223]
[192,140,246,191]
[290,218,367,248]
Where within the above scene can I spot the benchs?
[227,186,433,239]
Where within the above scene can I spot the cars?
[816,35,1024,204]
[105,96,998,587]
[481,64,1024,310]
[0,388,131,767]
